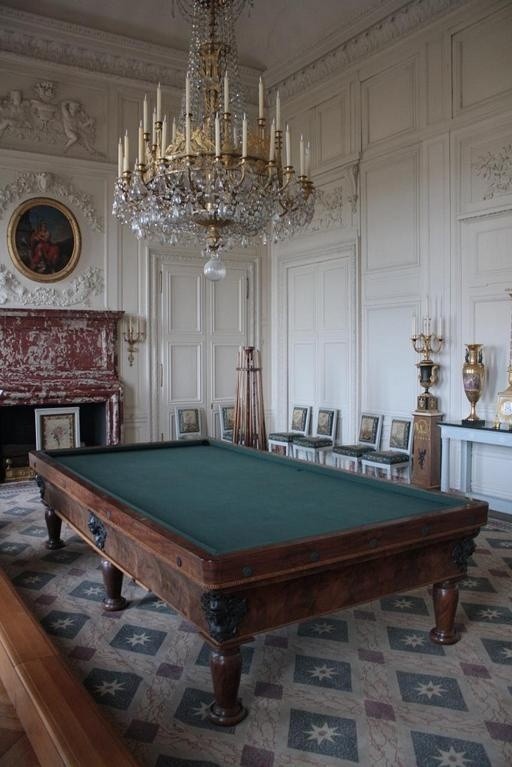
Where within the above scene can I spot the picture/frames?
[6,197,82,283]
[35,407,81,450]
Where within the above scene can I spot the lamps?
[110,0,317,281]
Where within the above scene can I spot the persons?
[29,220,52,272]
[1,90,33,132]
[61,100,97,155]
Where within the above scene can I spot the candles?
[129,316,132,332]
[411,308,416,336]
[437,295,442,338]
[137,316,140,333]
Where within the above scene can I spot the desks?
[437,420,512,493]
[29,437,488,727]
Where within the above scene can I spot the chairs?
[362,416,413,484]
[267,405,311,461]
[293,407,337,467]
[176,406,216,440]
[332,413,382,477]
[219,405,235,443]
